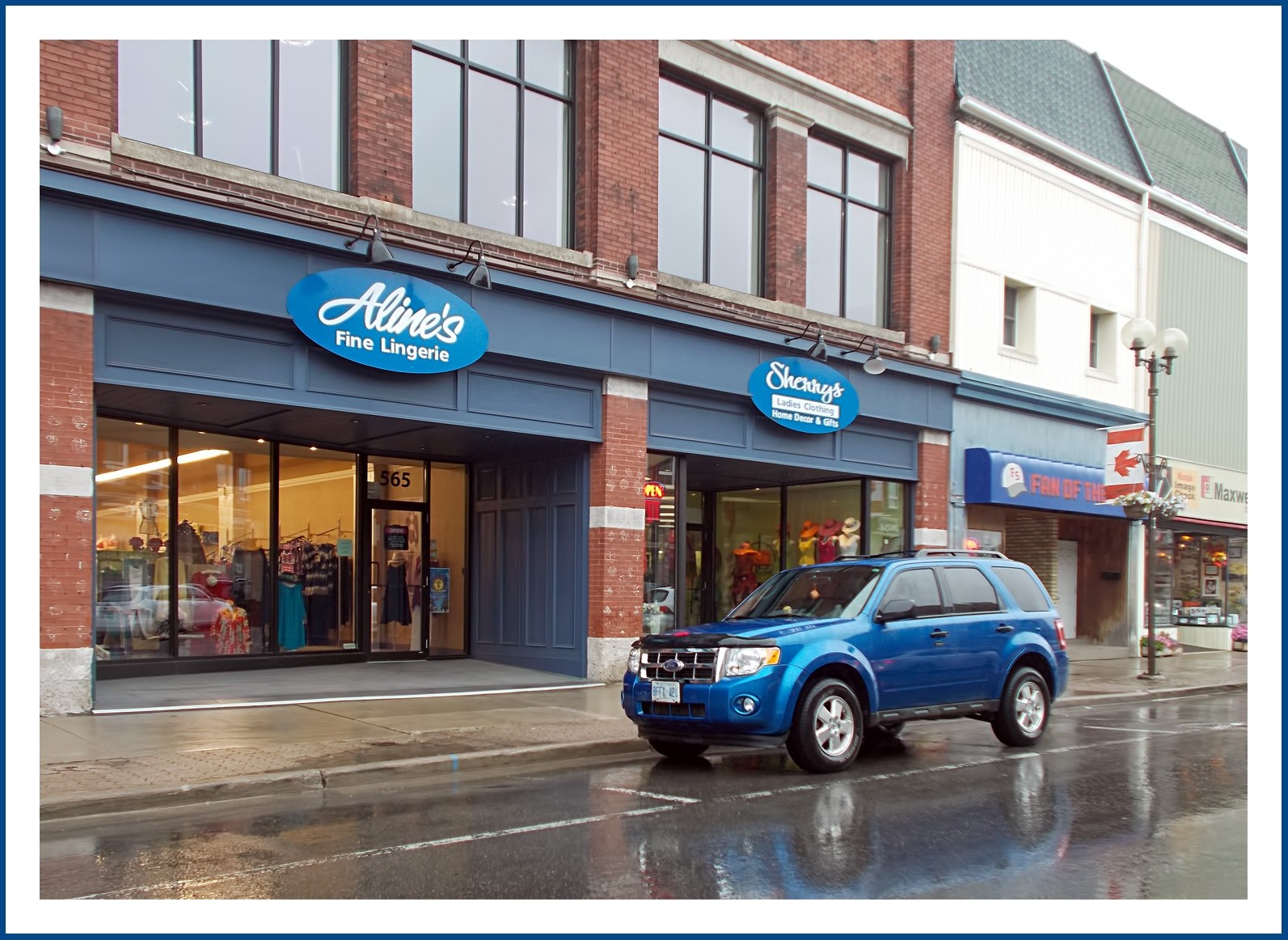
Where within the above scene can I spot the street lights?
[1121,314,1190,677]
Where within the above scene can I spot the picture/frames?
[1172,562,1240,626]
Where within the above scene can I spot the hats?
[842,517,861,532]
[800,522,819,538]
[387,552,408,562]
[818,519,841,536]
[733,543,761,555]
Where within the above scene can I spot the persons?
[771,524,796,573]
[798,520,820,565]
[817,519,842,563]
[734,541,762,604]
[839,517,861,560]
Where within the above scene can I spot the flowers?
[1231,623,1248,642]
[1140,633,1179,651]
[1115,490,1187,516]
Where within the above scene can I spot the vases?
[1231,641,1248,652]
[1157,509,1177,519]
[1124,504,1149,518]
[1142,646,1182,656]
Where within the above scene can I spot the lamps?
[46,106,63,142]
[344,214,393,265]
[784,322,828,361]
[446,240,491,289]
[931,335,939,354]
[628,255,639,280]
[841,334,886,375]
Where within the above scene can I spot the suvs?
[620,547,1073,776]
[95,583,248,642]
[642,585,674,630]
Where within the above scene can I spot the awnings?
[963,447,1147,520]
